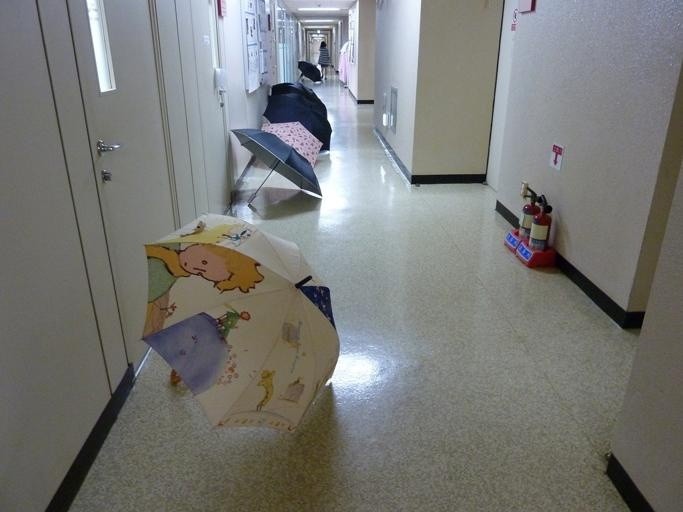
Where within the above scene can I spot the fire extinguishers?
[528,195,552,252]
[519,187,540,242]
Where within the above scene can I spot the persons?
[317,41,332,81]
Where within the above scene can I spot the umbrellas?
[137,212,341,436]
[230,129,322,204]
[270,81,327,120]
[262,93,332,151]
[259,121,323,168]
[297,61,322,84]
